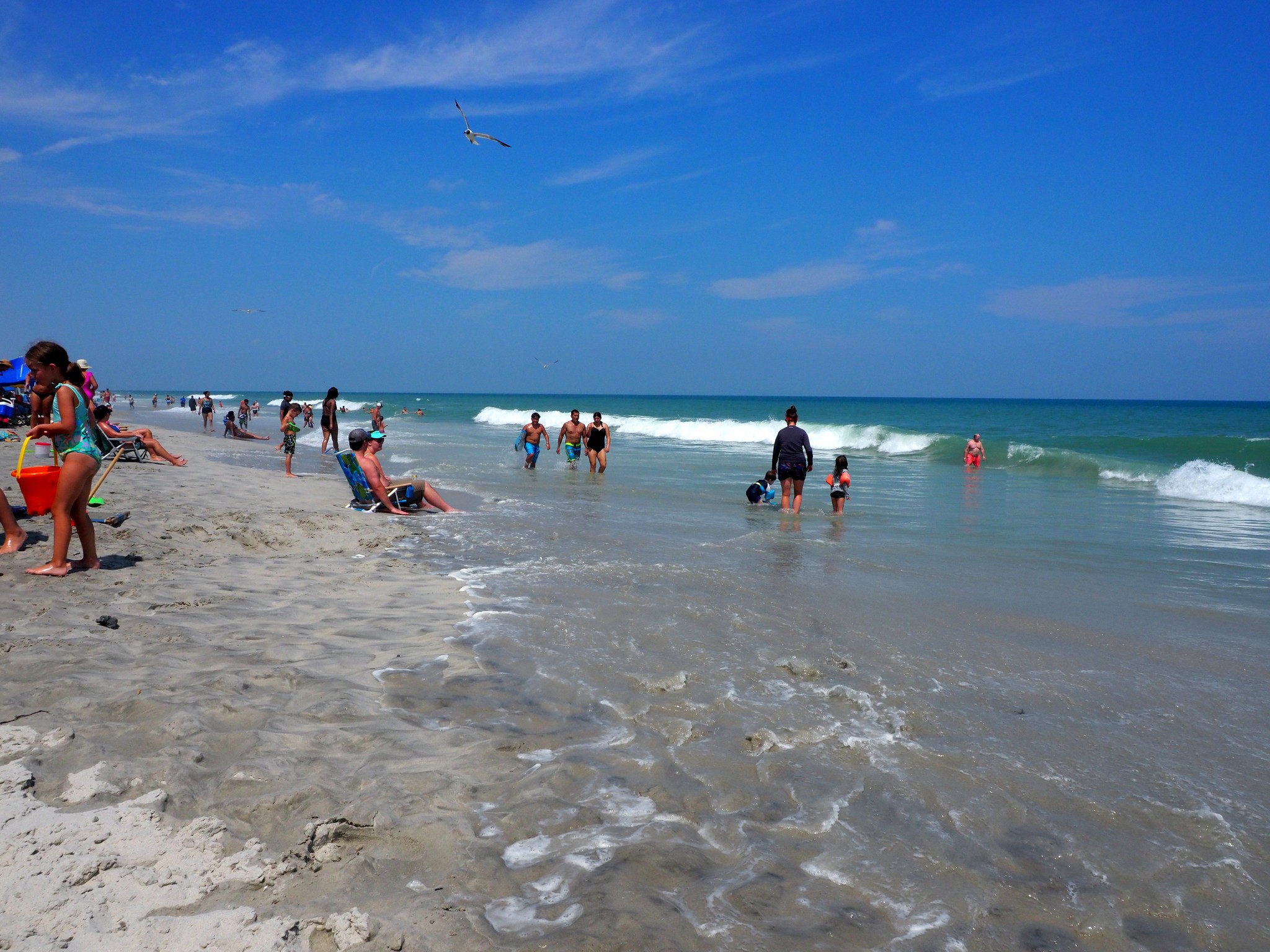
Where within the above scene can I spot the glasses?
[284,395,293,399]
[370,438,384,443]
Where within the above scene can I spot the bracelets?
[546,443,549,445]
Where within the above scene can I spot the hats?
[154,393,158,395]
[25,391,30,394]
[308,405,313,408]
[76,359,92,368]
[371,430,387,438]
[376,403,383,408]
[94,405,112,419]
[349,429,371,442]
[106,403,110,406]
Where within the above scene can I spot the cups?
[412,475,417,481]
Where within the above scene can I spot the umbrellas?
[0,355,35,391]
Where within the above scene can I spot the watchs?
[585,447,589,449]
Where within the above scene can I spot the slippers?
[95,615,118,628]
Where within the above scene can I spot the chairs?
[93,422,145,464]
[322,445,415,514]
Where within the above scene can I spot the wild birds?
[454,97,512,149]
[534,356,559,369]
[230,309,267,314]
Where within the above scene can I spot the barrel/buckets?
[35,442,51,458]
[12,431,61,516]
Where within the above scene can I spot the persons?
[339,406,351,413]
[964,433,986,467]
[522,413,550,468]
[0,488,27,555]
[24,340,103,576]
[746,471,777,504]
[189,391,270,440]
[302,402,315,428]
[321,387,339,454]
[771,406,813,514]
[413,409,424,415]
[587,412,611,473]
[556,409,589,464]
[369,408,373,412]
[363,431,392,480]
[363,407,366,411]
[401,407,408,413]
[372,402,388,433]
[349,428,461,514]
[275,391,302,477]
[0,360,189,466]
[830,455,851,512]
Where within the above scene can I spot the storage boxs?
[0,401,15,419]
